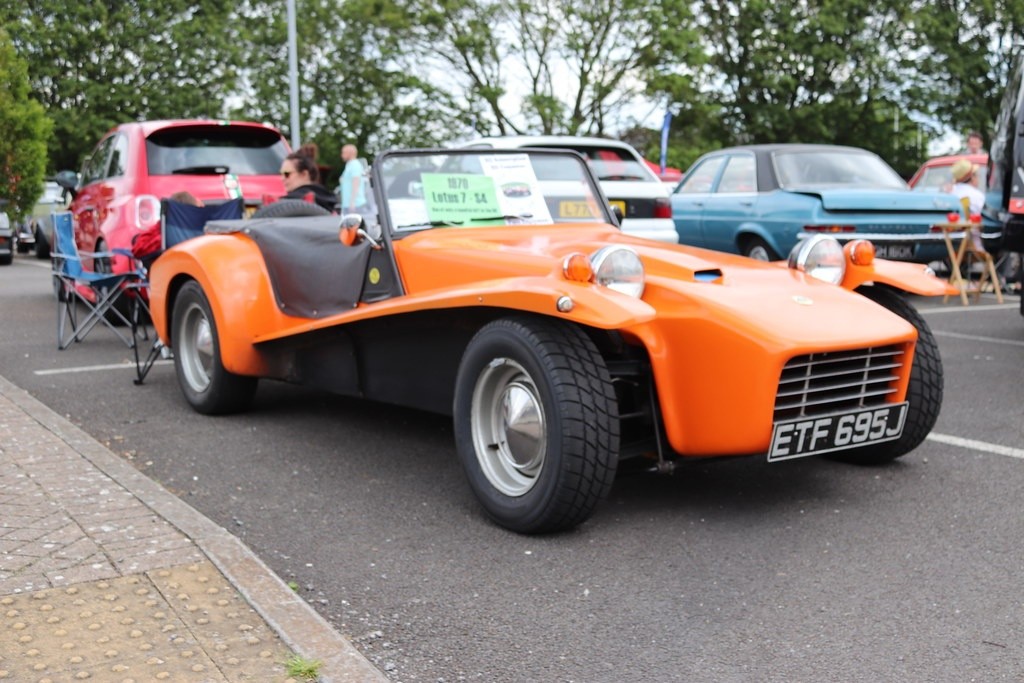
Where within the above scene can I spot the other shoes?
[155,339,174,358]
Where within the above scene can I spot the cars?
[386,133,678,243]
[149,140,946,536]
[16,179,70,256]
[910,154,1024,280]
[0,200,14,267]
[669,142,973,273]
[52,119,314,327]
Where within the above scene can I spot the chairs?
[111,197,245,386]
[968,223,1018,307]
[49,211,134,351]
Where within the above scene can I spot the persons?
[950,158,1015,282]
[980,189,1024,292]
[338,144,373,220]
[132,191,206,302]
[961,132,989,156]
[262,145,339,217]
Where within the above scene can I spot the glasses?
[280,171,297,178]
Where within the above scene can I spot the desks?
[932,220,976,306]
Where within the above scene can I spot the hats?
[952,159,980,183]
